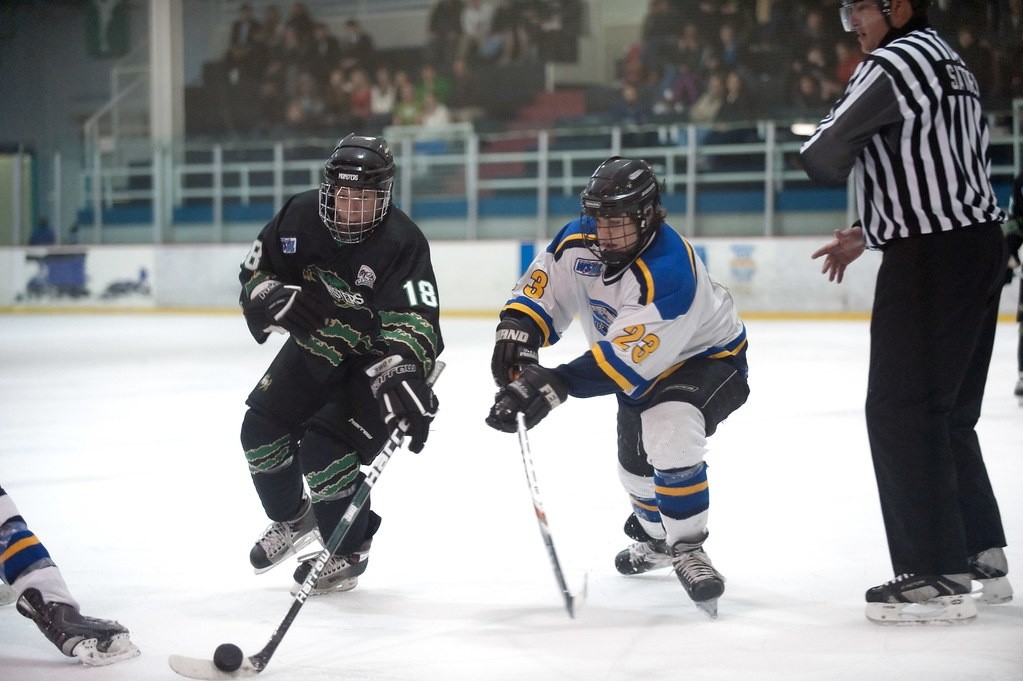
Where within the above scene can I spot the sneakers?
[248,494,320,575]
[668,528,725,619]
[17,586,141,668]
[968,547,1015,604]
[865,570,977,625]
[615,511,678,575]
[289,538,372,597]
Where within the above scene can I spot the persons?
[485,155,750,601]
[796,0,1010,604]
[1001,169,1023,397]
[182,0,1023,197]
[238,134,444,588]
[0,485,131,657]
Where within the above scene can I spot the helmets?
[579,156,663,285]
[839,0,895,31]
[320,132,396,243]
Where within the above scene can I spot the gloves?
[491,316,540,387]
[245,283,333,339]
[485,363,569,433]
[364,348,438,454]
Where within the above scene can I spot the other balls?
[212,643,244,672]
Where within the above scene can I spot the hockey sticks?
[512,365,650,628]
[167,358,447,681]
